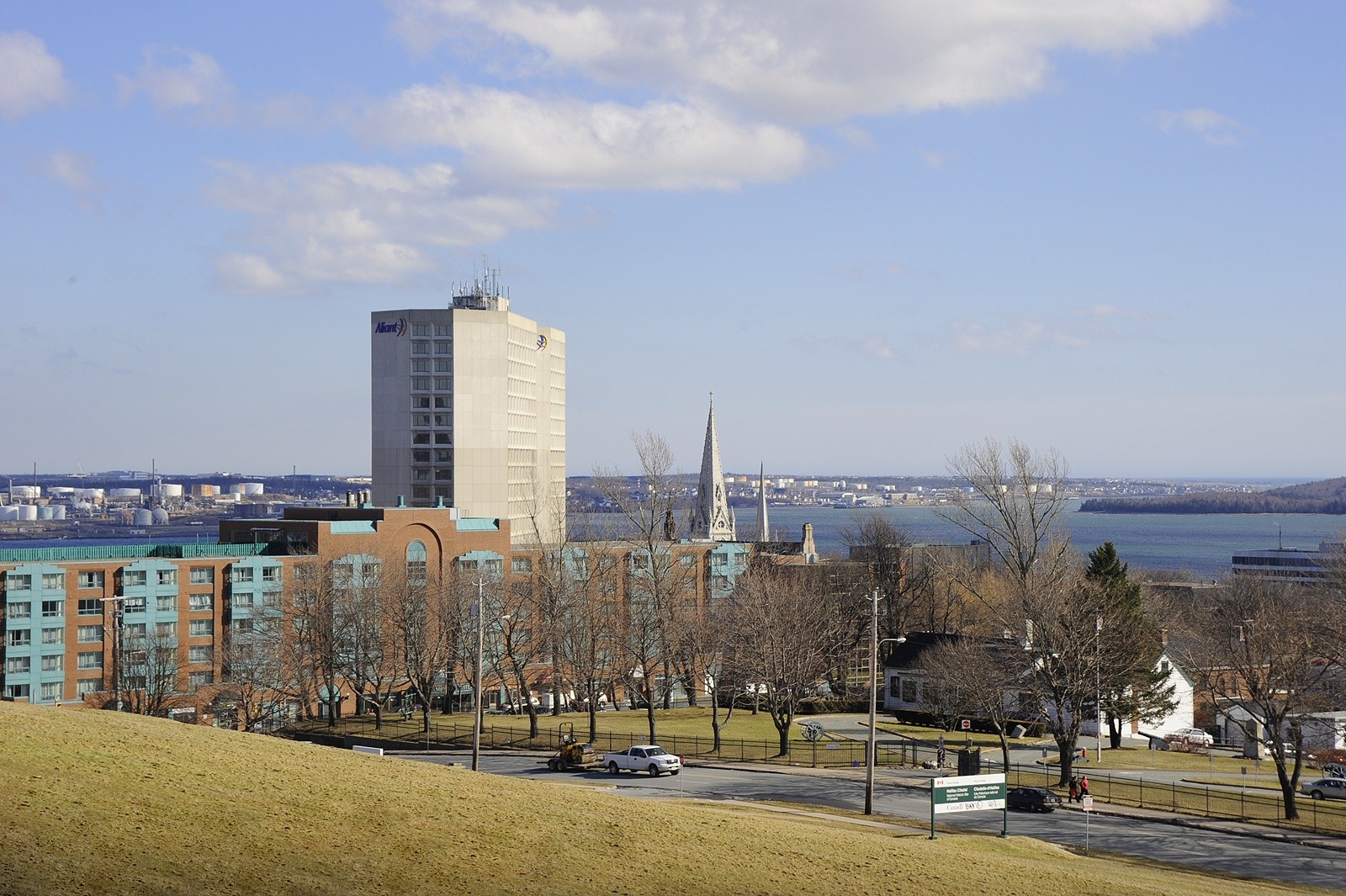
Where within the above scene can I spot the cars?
[1164,728,1214,748]
[1300,776,1346,800]
[1264,740,1306,758]
[1007,787,1063,813]
[390,704,423,711]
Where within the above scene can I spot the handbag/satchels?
[1069,788,1076,796]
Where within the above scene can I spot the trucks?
[541,693,572,709]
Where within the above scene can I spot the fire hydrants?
[1081,747,1086,758]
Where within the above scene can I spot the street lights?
[114,650,146,712]
[473,615,512,773]
[864,637,908,816]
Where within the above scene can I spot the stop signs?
[963,720,969,728]
[679,754,685,765]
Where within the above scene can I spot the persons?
[1078,775,1089,800]
[1068,775,1081,804]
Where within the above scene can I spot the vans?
[501,695,541,714]
[568,687,608,711]
[742,681,777,693]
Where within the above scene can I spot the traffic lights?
[55,702,61,707]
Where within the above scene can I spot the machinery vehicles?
[547,721,603,772]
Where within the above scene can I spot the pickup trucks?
[603,745,681,777]
